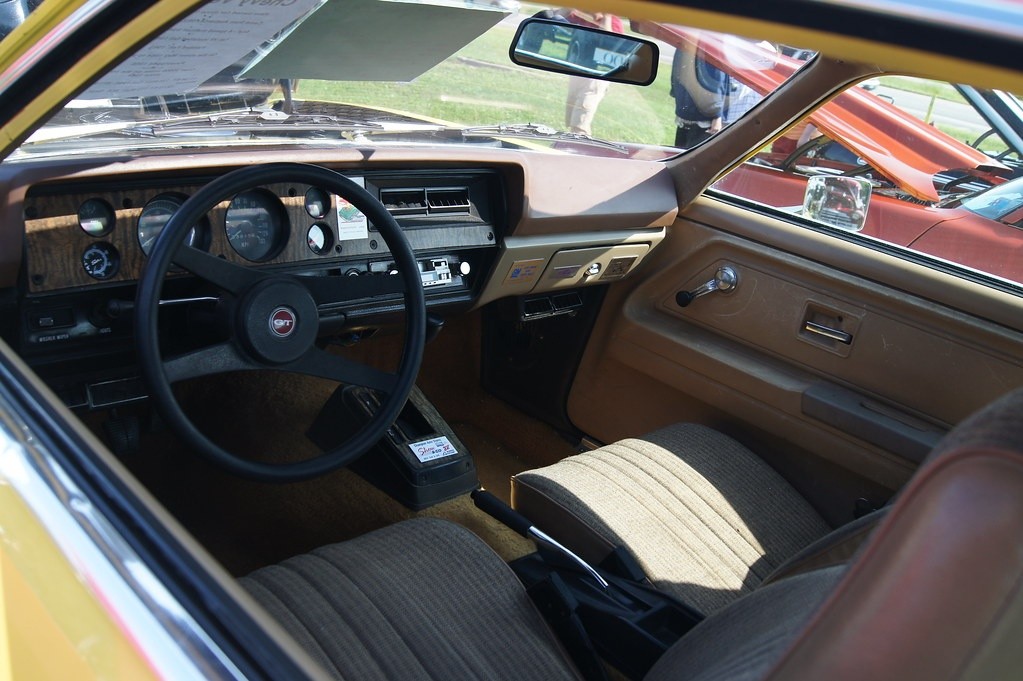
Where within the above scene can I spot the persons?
[726,34,822,152]
[671,30,733,147]
[562,9,624,136]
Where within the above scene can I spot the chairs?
[510,422,896,681]
[237,384,1023,681]
